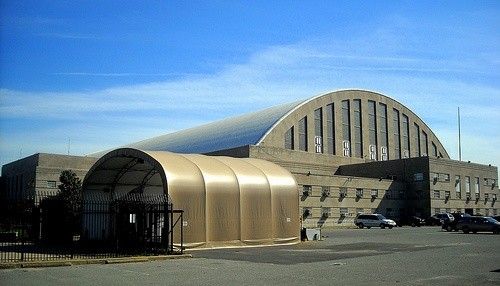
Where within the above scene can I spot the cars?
[420,216,441,226]
[397,216,426,227]
[456,216,500,234]
[442,215,466,232]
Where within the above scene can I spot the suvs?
[354,213,397,229]
[435,212,455,224]
